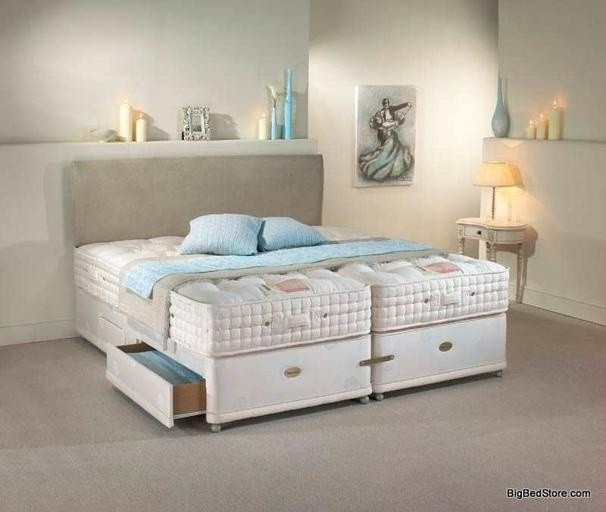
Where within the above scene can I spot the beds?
[69,154,512,437]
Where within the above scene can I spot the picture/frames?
[181,105,213,143]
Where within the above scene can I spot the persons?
[357,95,413,181]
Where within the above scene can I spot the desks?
[454,217,533,304]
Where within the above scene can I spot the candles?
[119,98,147,143]
[258,111,271,140]
[527,100,562,141]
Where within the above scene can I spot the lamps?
[472,160,524,225]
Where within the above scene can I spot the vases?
[491,78,509,138]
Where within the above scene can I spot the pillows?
[175,213,330,256]
